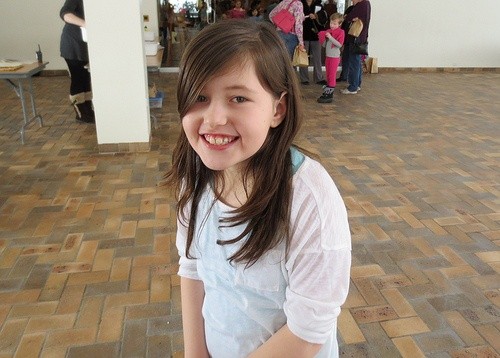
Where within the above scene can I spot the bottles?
[35,44,42,63]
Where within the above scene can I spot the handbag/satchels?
[271,0,296,34]
[348,19,363,37]
[292,46,309,67]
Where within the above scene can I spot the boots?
[69,91,95,122]
[318,85,335,103]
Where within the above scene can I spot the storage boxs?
[150,91,163,109]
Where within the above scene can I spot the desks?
[0,60,51,146]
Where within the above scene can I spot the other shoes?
[336,78,346,82]
[316,80,327,85]
[300,81,309,84]
[341,87,360,94]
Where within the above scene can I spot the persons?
[196,0,354,85]
[59,0,96,123]
[161,18,351,358]
[317,13,346,103]
[340,0,371,94]
